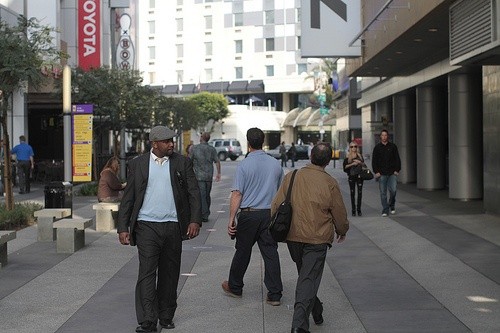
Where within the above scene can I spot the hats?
[149,126,178,142]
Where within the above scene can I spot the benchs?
[52,218,93,254]
[93,200,122,231]
[0,230,16,267]
[34,208,71,244]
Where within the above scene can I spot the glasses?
[349,145,357,148]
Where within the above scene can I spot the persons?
[117,126,202,333]
[289,143,296,167]
[188,132,221,223]
[222,128,285,306]
[98,157,127,203]
[280,142,287,167]
[344,141,364,217]
[11,136,35,194]
[372,130,401,216]
[271,143,348,333]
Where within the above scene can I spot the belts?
[241,208,266,211]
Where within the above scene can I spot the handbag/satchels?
[358,169,374,180]
[265,200,292,242]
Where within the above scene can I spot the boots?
[352,205,356,216]
[357,204,362,216]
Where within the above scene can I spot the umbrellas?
[245,96,261,103]
[223,96,235,103]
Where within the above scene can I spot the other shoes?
[203,218,209,223]
[313,302,324,324]
[136,322,162,333]
[291,327,312,333]
[382,208,389,217]
[266,298,281,306]
[222,281,242,298]
[390,208,396,215]
[159,312,176,329]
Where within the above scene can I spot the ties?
[155,158,167,166]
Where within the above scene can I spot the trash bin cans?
[44,180,73,220]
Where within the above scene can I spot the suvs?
[209,138,242,161]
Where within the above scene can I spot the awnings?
[229,80,264,91]
[180,85,195,93]
[196,82,229,91]
[163,85,178,94]
[282,107,337,126]
[148,86,162,94]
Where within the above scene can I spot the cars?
[265,144,340,161]
